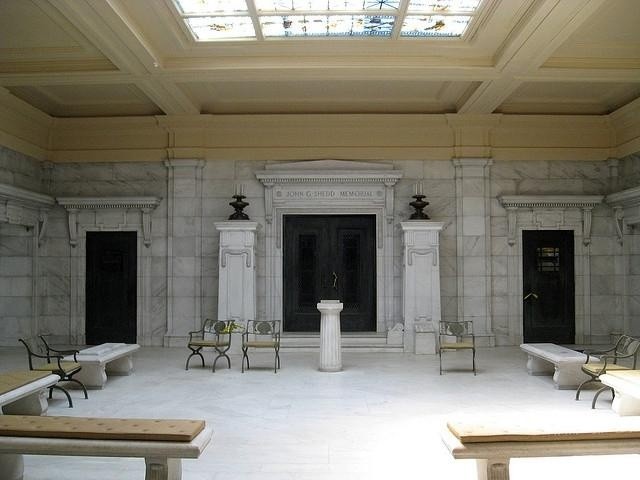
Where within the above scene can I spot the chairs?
[241,320,281,373]
[575,335,640,410]
[18,335,88,408]
[185,318,235,372]
[438,320,477,375]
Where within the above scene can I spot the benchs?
[519,343,600,390]
[0,416,213,480]
[441,415,640,480]
[61,343,140,390]
[0,371,61,416]
[600,370,640,413]
